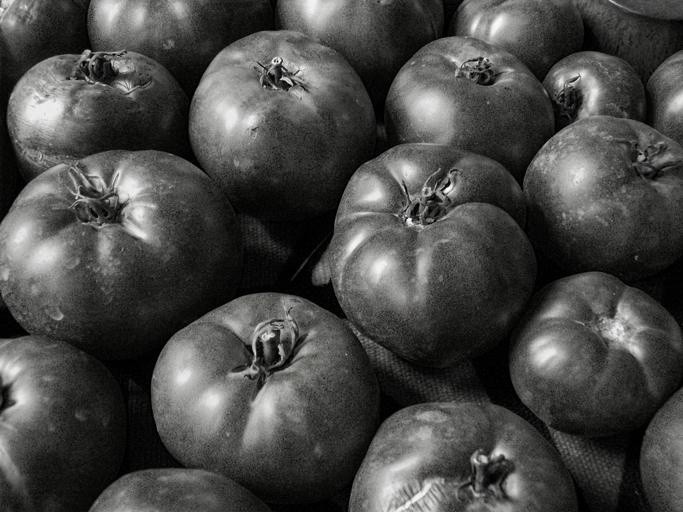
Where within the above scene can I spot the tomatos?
[0,1,683,512]
